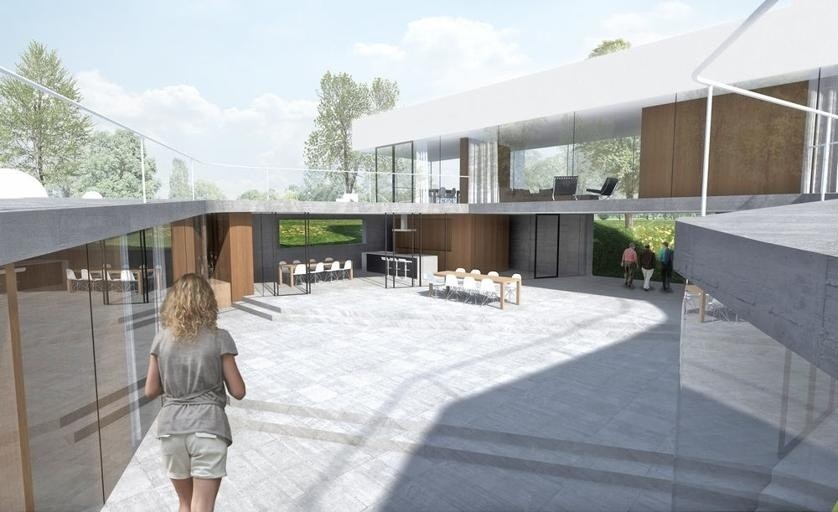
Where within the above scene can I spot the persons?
[144,273,244,510]
[638,243,656,291]
[658,242,673,290]
[620,241,639,289]
